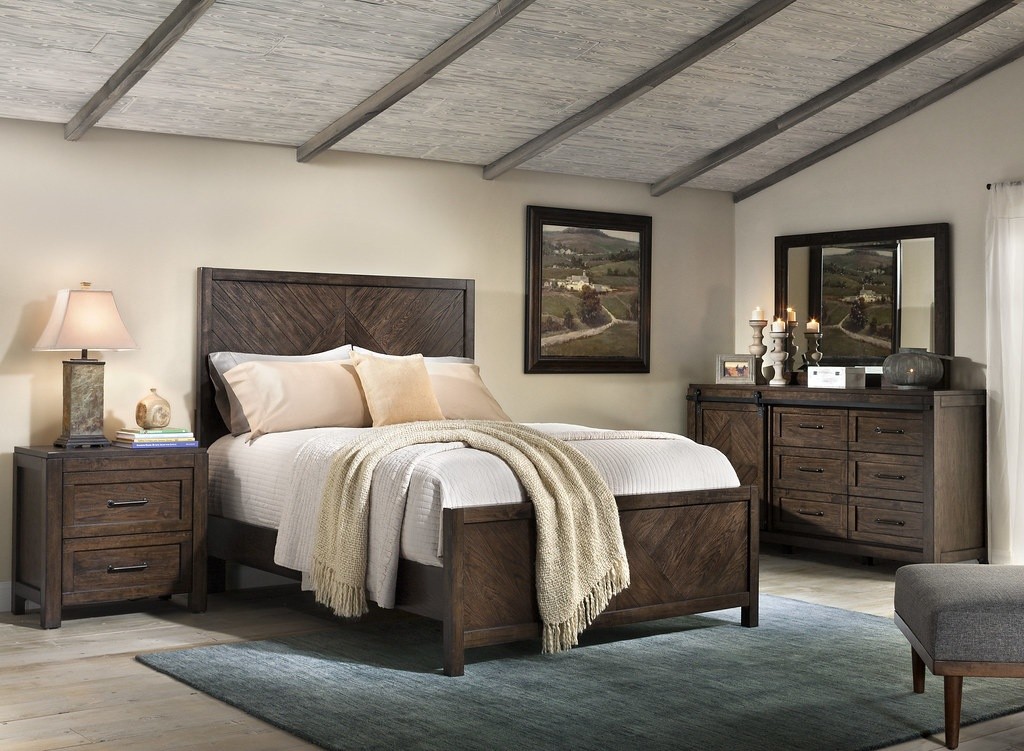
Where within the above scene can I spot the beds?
[195,268,761,677]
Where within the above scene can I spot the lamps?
[31,281,137,449]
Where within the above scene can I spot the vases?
[136,388,171,431]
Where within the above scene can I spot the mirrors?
[773,222,950,388]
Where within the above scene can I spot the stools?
[894,565,1024,750]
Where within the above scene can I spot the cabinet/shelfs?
[686,383,986,565]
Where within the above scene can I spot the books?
[110,428,198,448]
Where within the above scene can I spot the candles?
[807,318,819,333]
[752,306,765,320]
[787,308,796,321]
[773,317,785,332]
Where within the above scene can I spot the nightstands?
[11,445,210,629]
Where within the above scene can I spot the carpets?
[133,589,1024,751]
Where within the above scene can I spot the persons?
[736,364,739,376]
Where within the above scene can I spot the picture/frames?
[808,239,901,366]
[525,204,652,374]
[716,354,756,385]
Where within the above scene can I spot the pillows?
[207,343,512,442]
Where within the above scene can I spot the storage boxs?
[807,366,865,390]
